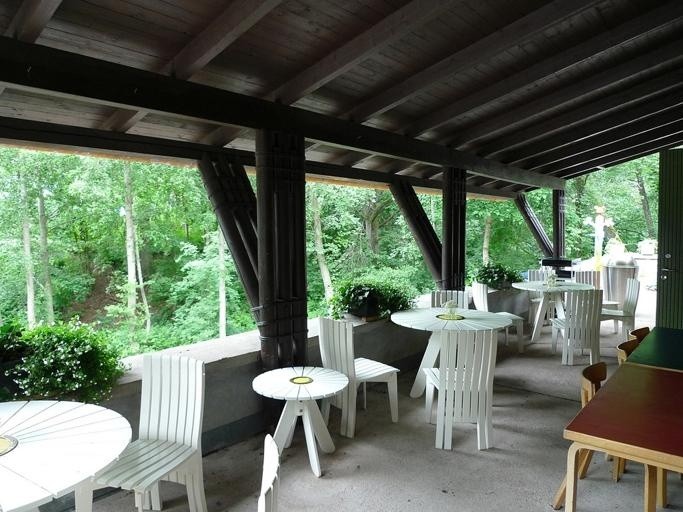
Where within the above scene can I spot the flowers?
[5,318,132,406]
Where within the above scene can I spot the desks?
[627,326,683,370]
[0,399,133,512]
[253,367,349,477]
[563,363,683,512]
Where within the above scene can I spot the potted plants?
[477,264,523,292]
[638,240,658,254]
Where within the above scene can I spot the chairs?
[75,355,208,512]
[259,434,280,512]
